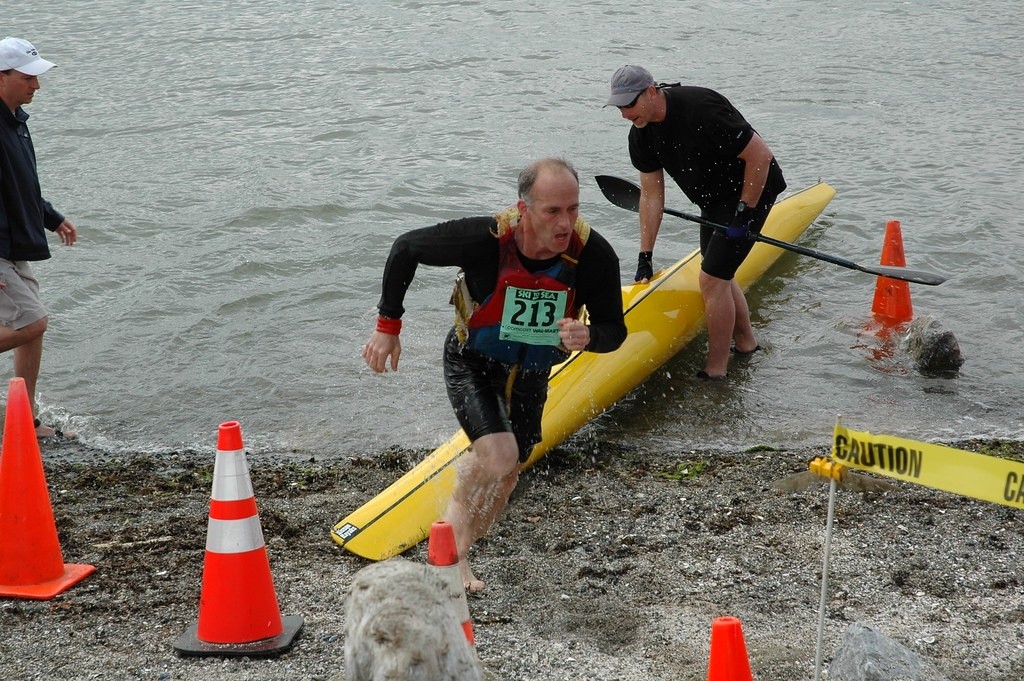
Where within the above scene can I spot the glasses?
[615,89,646,108]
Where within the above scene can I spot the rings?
[569,335,573,342]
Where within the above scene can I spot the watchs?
[736,200,757,215]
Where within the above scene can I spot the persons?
[0,36,78,439]
[601,64,788,380]
[361,158,628,591]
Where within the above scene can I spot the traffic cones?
[870,220,914,324]
[706,616,755,681]
[1,378,94,599]
[174,420,305,658]
[425,522,479,667]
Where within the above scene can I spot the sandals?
[34,418,76,441]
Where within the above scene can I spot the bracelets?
[376,316,402,336]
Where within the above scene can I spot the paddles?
[593,172,951,288]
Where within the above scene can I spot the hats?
[0,36,58,75]
[602,65,654,108]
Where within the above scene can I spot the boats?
[327,183,836,563]
